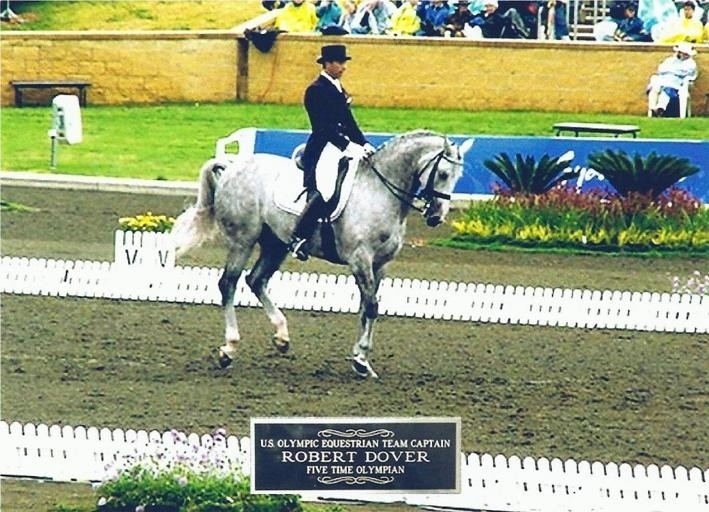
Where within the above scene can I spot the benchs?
[8,80,92,108]
[551,122,640,138]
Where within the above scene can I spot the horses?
[172,128,475,379]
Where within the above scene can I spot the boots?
[288,189,327,261]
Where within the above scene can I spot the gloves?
[363,142,376,155]
[345,141,369,161]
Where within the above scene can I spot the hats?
[316,45,351,64]
[673,42,698,56]
[453,0,471,6]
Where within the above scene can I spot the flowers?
[118,210,177,232]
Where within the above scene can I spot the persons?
[287,42,381,263]
[645,40,698,116]
